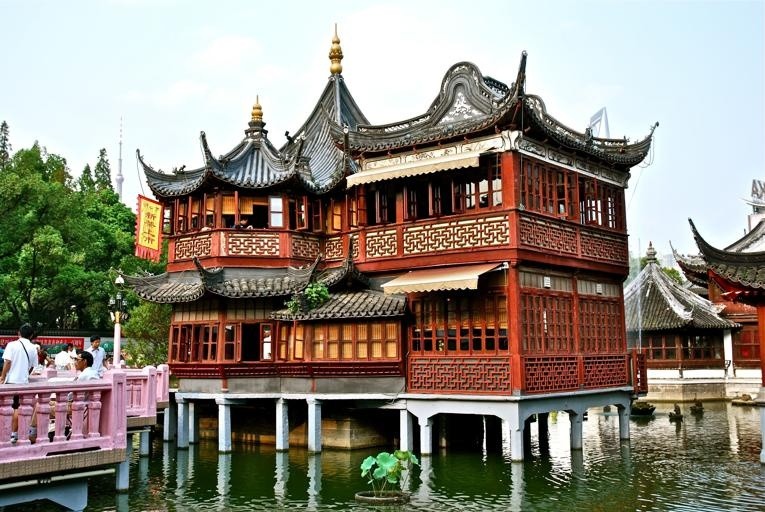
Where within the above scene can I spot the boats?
[732,399,754,405]
[603,406,611,412]
[691,411,705,415]
[631,405,656,415]
[731,405,755,407]
[690,405,705,411]
[670,418,683,423]
[629,415,656,421]
[604,414,610,421]
[668,412,684,418]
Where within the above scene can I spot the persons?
[176,216,252,235]
[0,320,146,444]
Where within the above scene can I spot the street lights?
[108,274,130,367]
